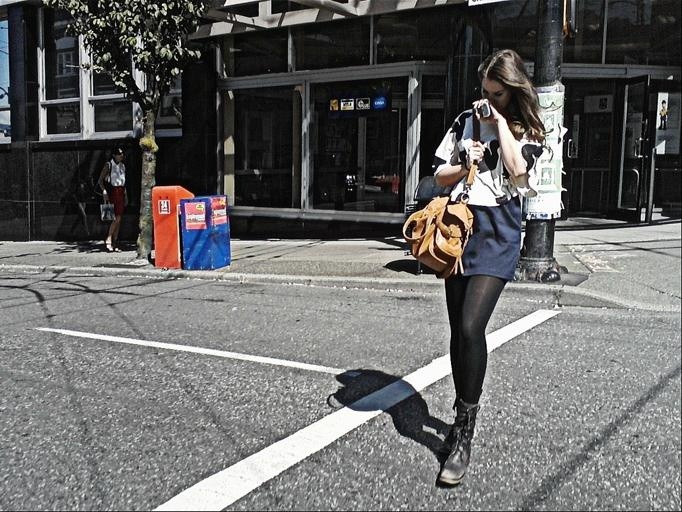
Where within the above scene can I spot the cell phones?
[478,99,492,118]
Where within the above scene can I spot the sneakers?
[104,241,122,253]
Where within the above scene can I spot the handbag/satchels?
[93,180,113,196]
[402,197,475,278]
[100,199,117,221]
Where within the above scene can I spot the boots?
[432,416,456,453]
[437,399,480,485]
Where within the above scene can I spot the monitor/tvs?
[355,96,371,112]
[330,99,339,112]
[371,95,390,111]
[340,98,355,112]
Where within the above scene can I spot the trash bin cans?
[414,176,457,273]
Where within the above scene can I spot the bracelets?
[461,160,470,172]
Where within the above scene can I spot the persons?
[67,162,105,244]
[96,147,131,253]
[432,45,558,491]
